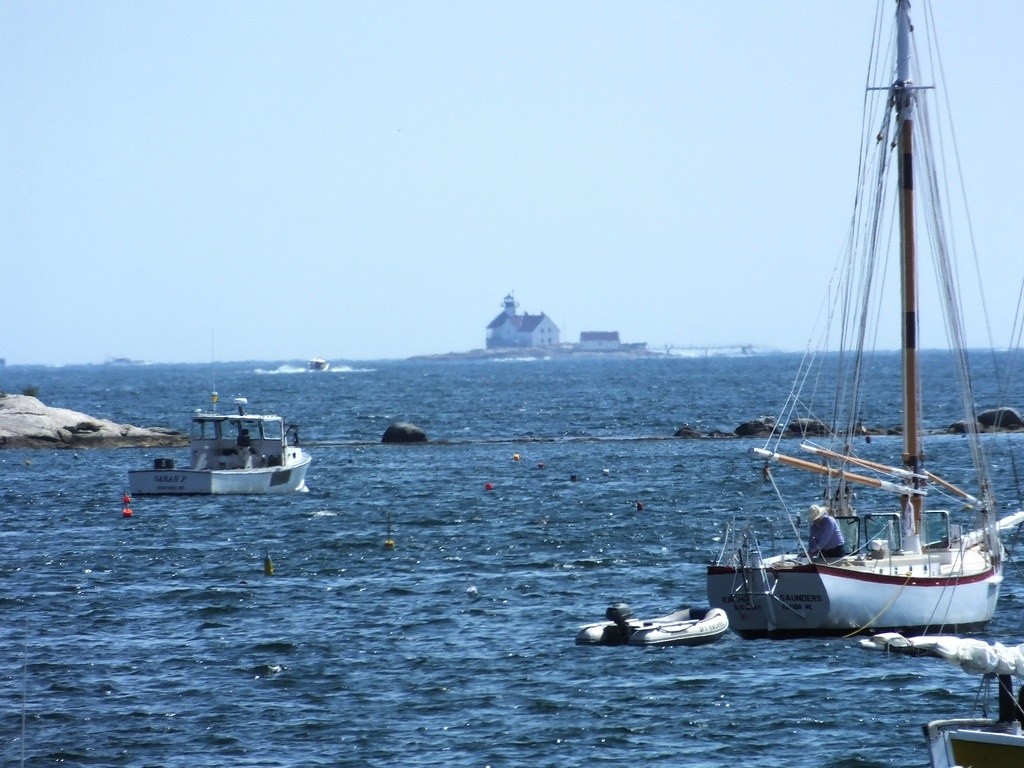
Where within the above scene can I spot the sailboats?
[707,0,1024,640]
[857,276,1024,768]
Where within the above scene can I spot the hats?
[806,505,826,523]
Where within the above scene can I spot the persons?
[797,505,845,559]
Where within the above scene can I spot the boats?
[575,602,729,647]
[307,358,330,373]
[128,328,312,495]
